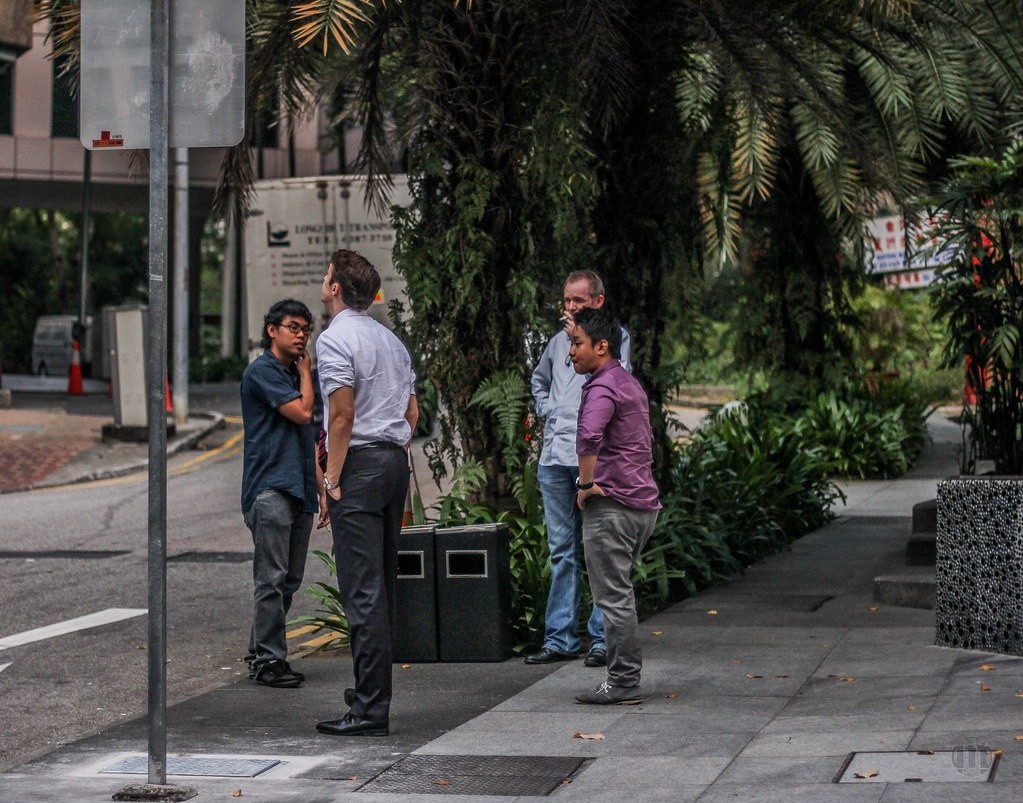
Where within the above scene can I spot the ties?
[318,419,327,474]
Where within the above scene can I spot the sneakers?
[574,681,644,705]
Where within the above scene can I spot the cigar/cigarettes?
[558,316,568,321]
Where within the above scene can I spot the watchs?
[322,473,340,490]
[576,477,594,490]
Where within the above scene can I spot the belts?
[348,441,399,453]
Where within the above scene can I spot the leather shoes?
[247,661,306,681]
[315,710,389,736]
[523,647,579,664]
[254,659,301,687]
[583,648,607,666]
[343,687,356,707]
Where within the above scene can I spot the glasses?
[277,322,313,336]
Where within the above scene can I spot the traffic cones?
[165,371,173,416]
[64,341,87,396]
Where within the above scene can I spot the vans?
[29,314,92,378]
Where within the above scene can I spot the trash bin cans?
[435,522,515,662]
[393,524,446,664]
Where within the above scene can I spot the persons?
[314,250,419,736]
[524,269,632,666]
[239,297,330,685]
[568,307,660,705]
